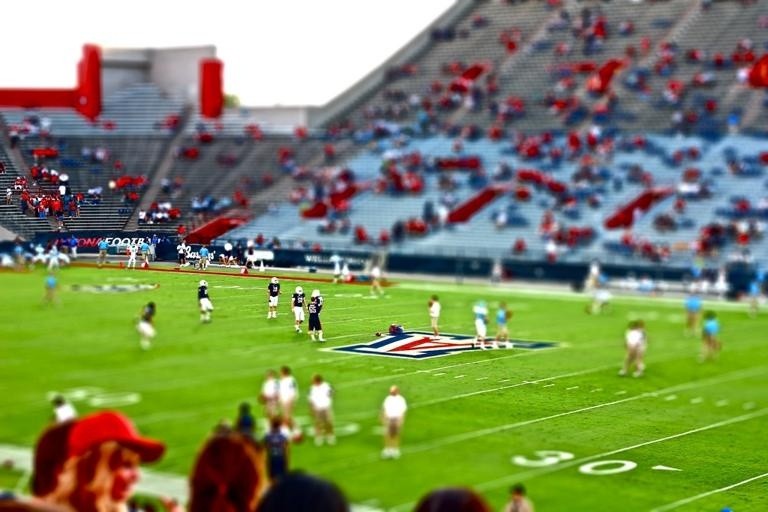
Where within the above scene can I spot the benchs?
[0,1,766,294]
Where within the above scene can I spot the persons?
[1,104,767,379]
[364,0,767,140]
[1,363,534,512]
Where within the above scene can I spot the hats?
[66,412,166,463]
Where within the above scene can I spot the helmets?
[272,277,278,284]
[296,287,303,295]
[201,281,207,286]
[311,290,320,297]
